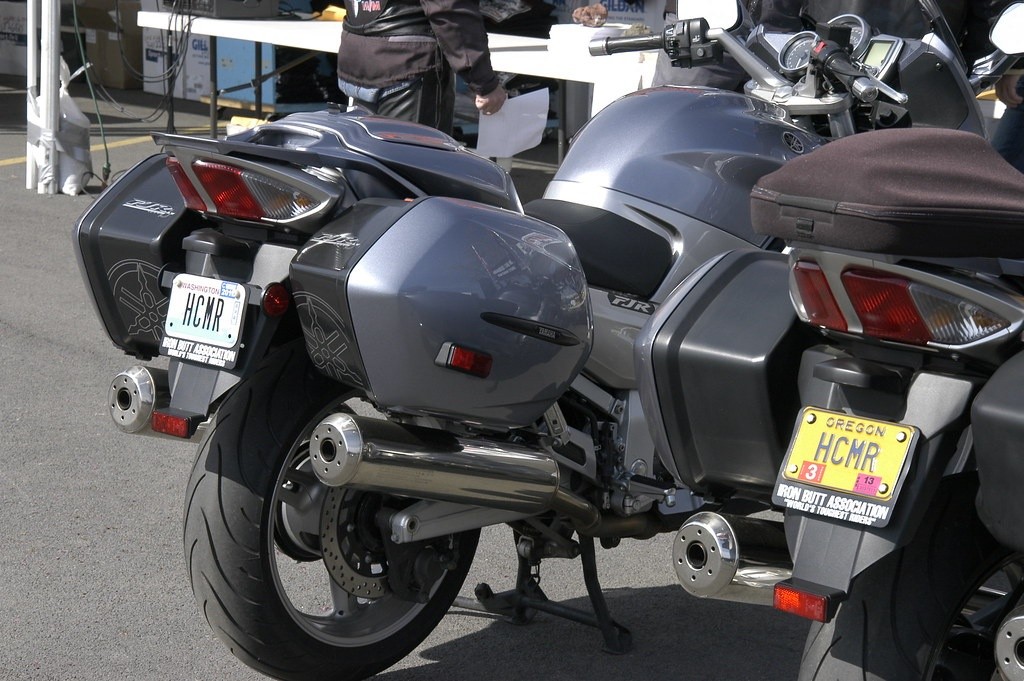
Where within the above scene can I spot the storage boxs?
[69,0,143,90]
[139,0,279,101]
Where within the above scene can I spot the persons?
[339,0,507,136]
[650,0,1024,170]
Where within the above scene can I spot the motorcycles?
[635,1,1024,681]
[73,0,995,681]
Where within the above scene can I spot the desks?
[137,11,668,176]
